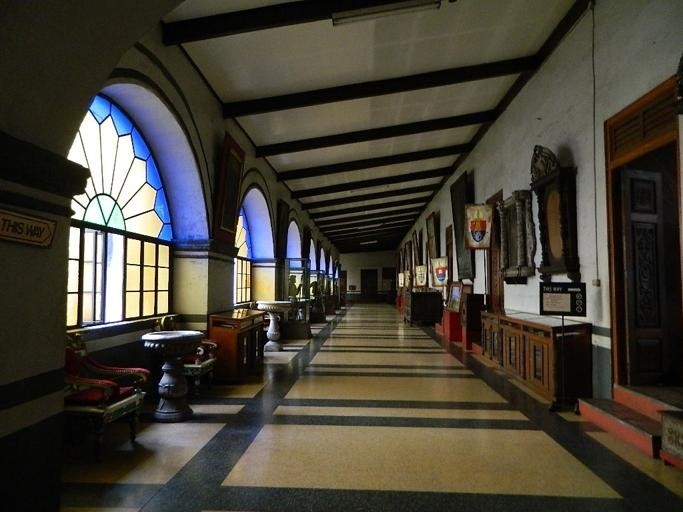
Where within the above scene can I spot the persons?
[287,275,300,296]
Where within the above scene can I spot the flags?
[414,265,427,287]
[403,271,411,288]
[398,273,405,288]
[464,205,493,250]
[430,256,448,287]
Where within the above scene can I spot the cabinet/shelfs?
[479,311,501,364]
[404,291,441,327]
[499,313,592,399]
[207,308,266,380]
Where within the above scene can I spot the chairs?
[62,334,150,445]
[161,313,217,395]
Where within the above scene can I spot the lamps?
[330,0,442,26]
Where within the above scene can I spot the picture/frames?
[495,189,534,280]
[447,283,463,312]
